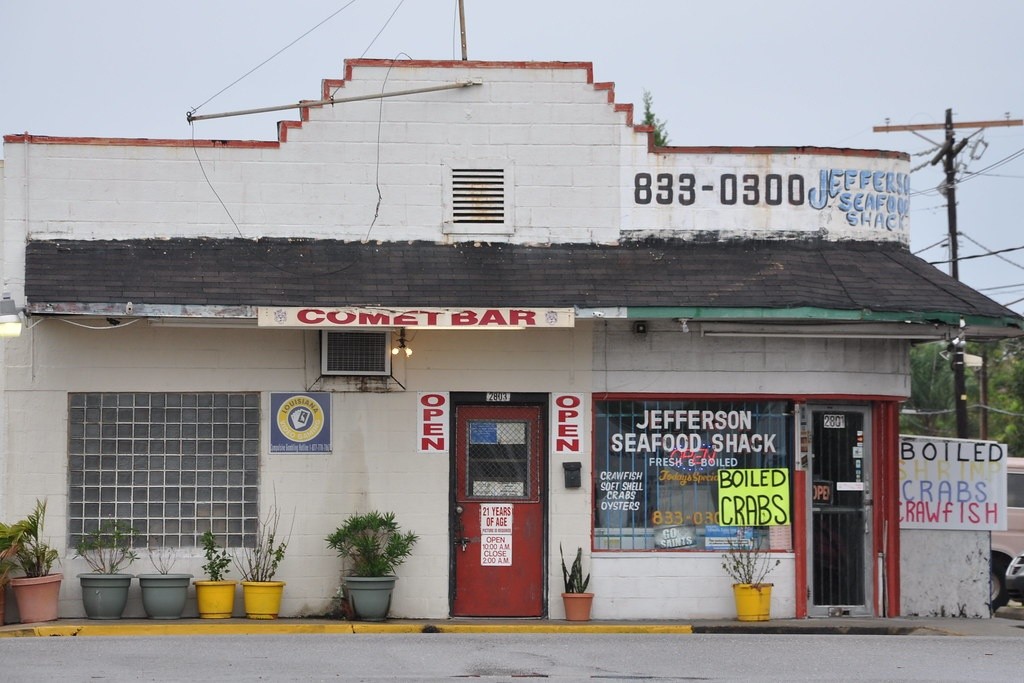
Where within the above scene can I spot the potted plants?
[559,542,594,621]
[0,522,9,626]
[9,497,65,624]
[232,480,297,620]
[721,524,780,621]
[324,509,421,622]
[136,537,194,619]
[72,518,140,619]
[193,530,239,619]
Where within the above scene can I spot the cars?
[990,457,1024,613]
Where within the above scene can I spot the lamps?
[391,330,412,357]
[0,297,26,339]
